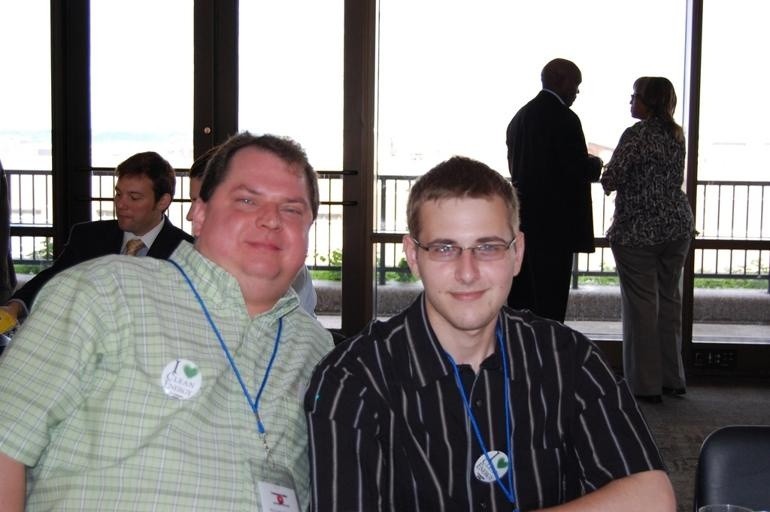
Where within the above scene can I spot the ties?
[125,239,147,256]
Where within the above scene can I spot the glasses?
[409,236,518,261]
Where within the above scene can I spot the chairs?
[696,426,770,510]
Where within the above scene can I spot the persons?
[0,151,194,337]
[505,57,603,325]
[303,157,677,510]
[0,130,337,512]
[601,76,697,407]
